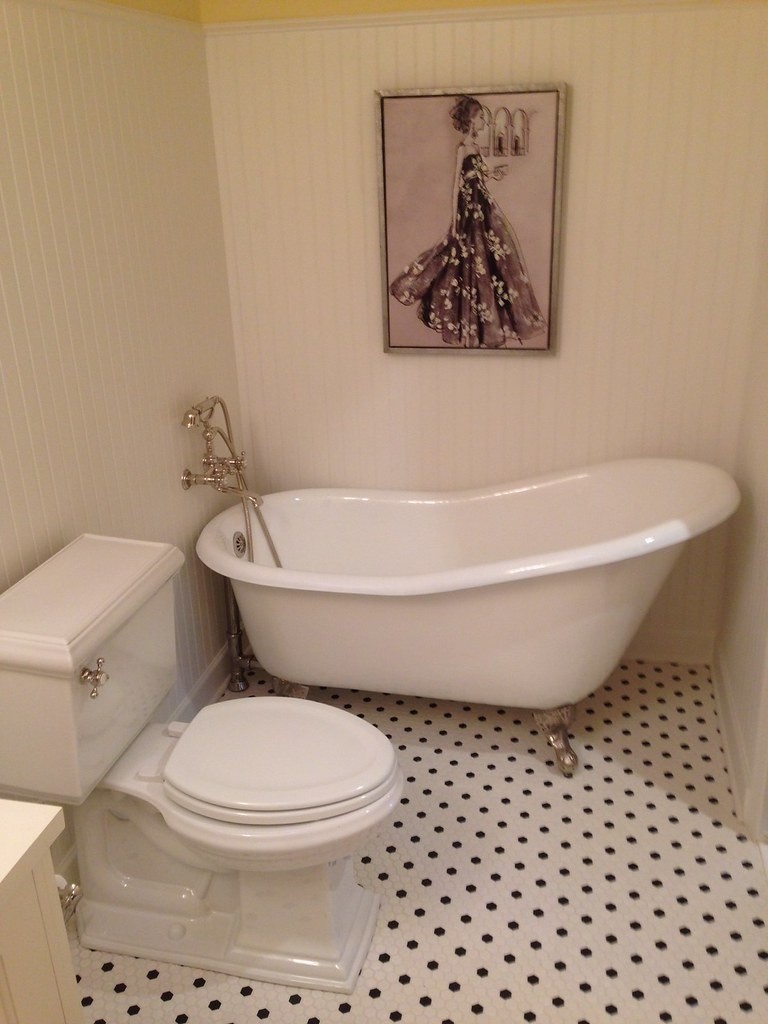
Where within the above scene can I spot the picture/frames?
[373,82,567,357]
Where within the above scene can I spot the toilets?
[2,529,399,997]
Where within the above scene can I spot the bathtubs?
[196,455,740,780]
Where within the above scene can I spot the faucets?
[203,454,264,508]
